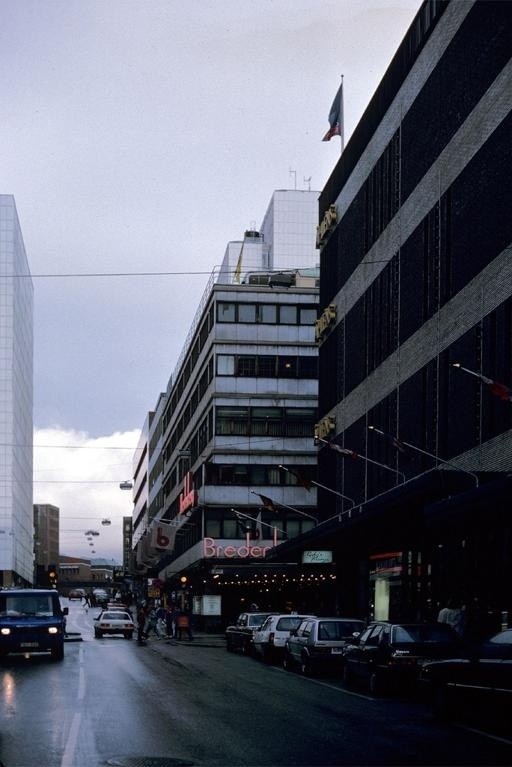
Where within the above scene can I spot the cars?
[92,589,135,640]
[251,614,319,658]
[282,616,369,674]
[68,589,83,601]
[0,587,69,662]
[341,620,466,694]
[415,647,510,733]
[225,611,280,655]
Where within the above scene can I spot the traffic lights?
[48,572,58,588]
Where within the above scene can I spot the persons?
[112,588,194,643]
[82,593,91,608]
[437,596,469,633]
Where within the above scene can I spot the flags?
[320,82,342,141]
